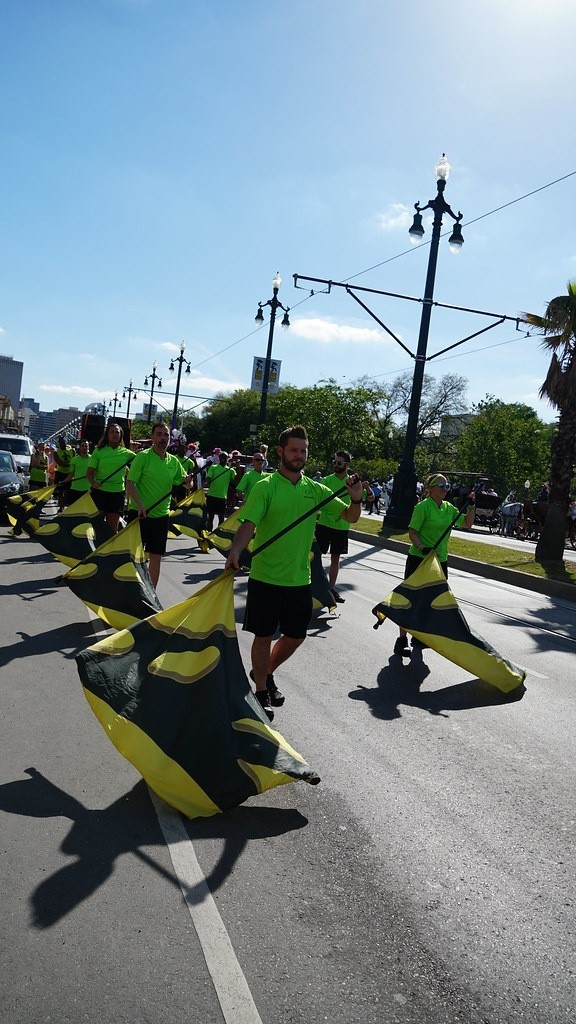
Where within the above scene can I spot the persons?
[130,439,269,505]
[237,454,271,506]
[394,474,475,656]
[87,423,143,531]
[225,426,363,719]
[126,423,193,590]
[315,451,375,602]
[312,472,322,482]
[203,452,240,533]
[414,482,551,539]
[366,483,393,515]
[30,437,92,515]
[174,444,199,498]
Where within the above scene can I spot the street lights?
[255,271,291,433]
[122,377,137,420]
[382,152,467,531]
[108,389,122,417]
[168,338,191,437]
[68,397,109,442]
[144,358,163,427]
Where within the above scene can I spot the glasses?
[332,460,347,466]
[432,481,450,489]
[255,458,264,462]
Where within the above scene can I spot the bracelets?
[351,497,362,504]
[366,491,373,496]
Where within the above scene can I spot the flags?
[310,536,337,614]
[206,508,257,569]
[167,488,212,552]
[65,520,164,632]
[33,493,116,570]
[77,569,321,819]
[6,485,56,535]
[373,549,527,694]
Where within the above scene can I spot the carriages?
[381,470,576,552]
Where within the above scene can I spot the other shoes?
[58,507,65,513]
[395,637,411,656]
[250,670,285,707]
[411,637,429,648]
[331,590,345,604]
[254,690,274,721]
[369,510,373,515]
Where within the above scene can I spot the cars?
[0,450,27,519]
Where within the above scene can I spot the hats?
[316,471,321,475]
[219,451,229,457]
[213,448,221,453]
[188,443,196,449]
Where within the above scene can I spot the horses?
[501,492,524,538]
[379,478,394,511]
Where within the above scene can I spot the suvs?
[0,432,36,492]
